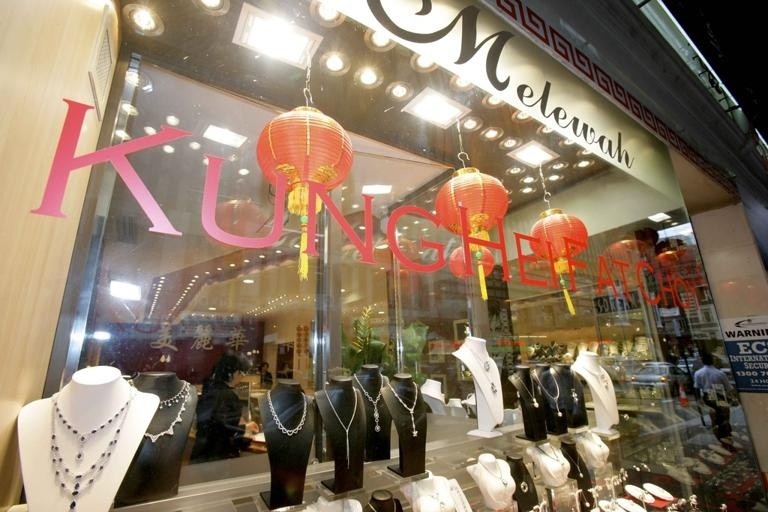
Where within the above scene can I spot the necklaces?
[267,373,418,470]
[368,499,396,512]
[465,343,609,417]
[47,381,191,511]
[415,433,602,512]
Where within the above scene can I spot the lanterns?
[255,106,351,282]
[435,166,687,316]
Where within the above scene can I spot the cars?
[632,357,703,395]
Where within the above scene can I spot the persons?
[258,361,273,389]
[693,350,739,449]
[189,356,259,464]
